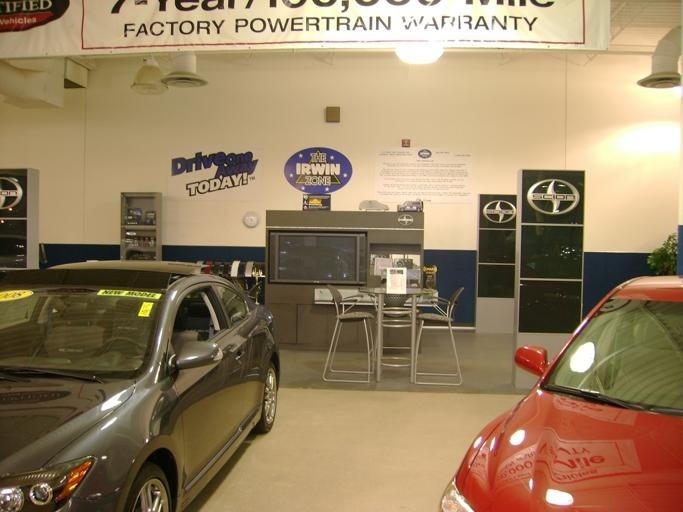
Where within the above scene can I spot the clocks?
[242,211,260,228]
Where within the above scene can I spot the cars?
[438,274,683,512]
[0,260,281,512]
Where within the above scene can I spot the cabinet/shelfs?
[120,192,163,263]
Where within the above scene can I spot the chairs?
[610,315,683,409]
[413,286,466,386]
[43,303,104,360]
[321,284,375,383]
[186,302,210,329]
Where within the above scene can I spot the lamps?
[130,55,167,95]
[161,52,209,89]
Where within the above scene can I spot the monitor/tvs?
[269,232,366,286]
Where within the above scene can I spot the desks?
[359,287,437,382]
